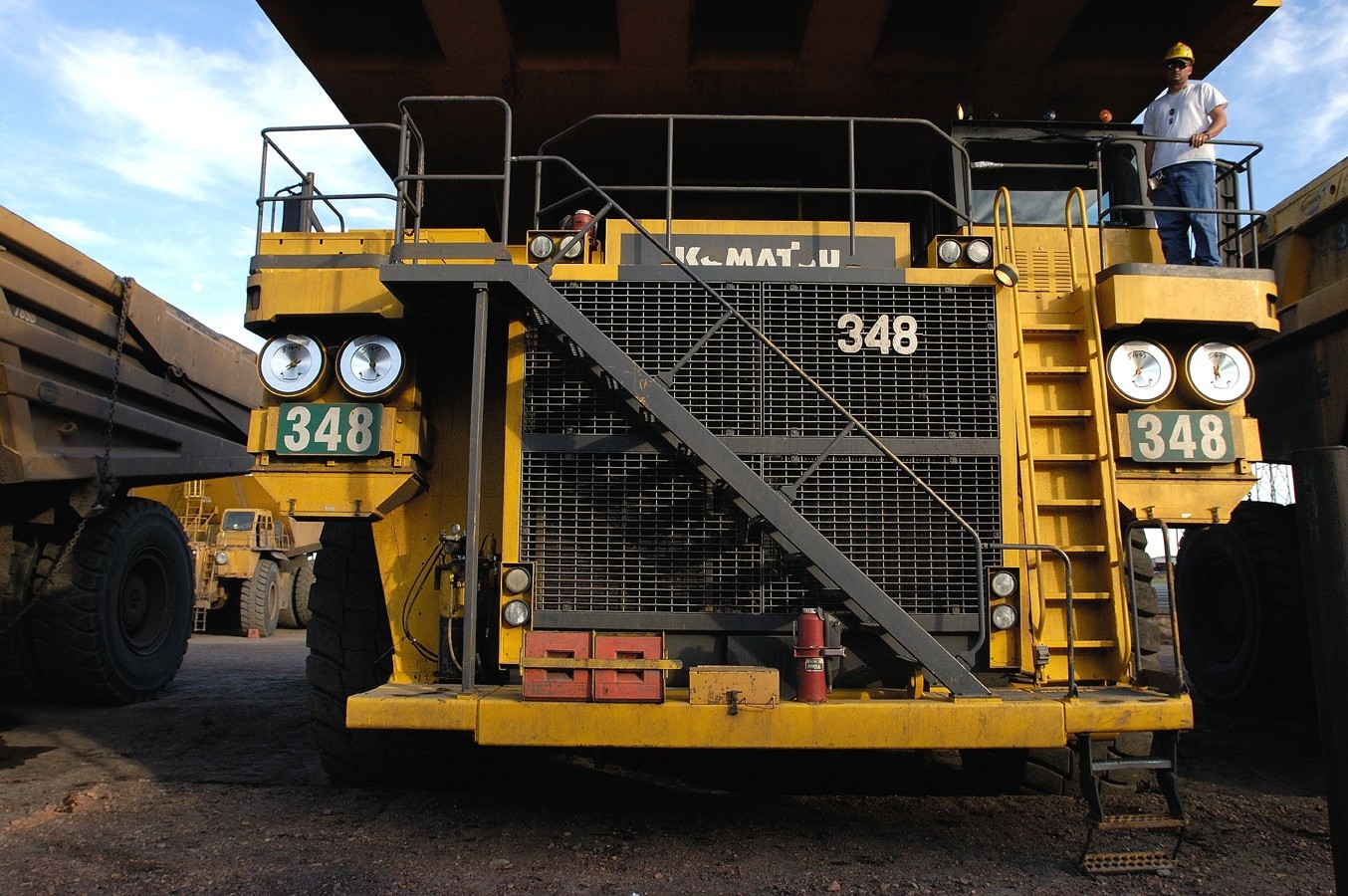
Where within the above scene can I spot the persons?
[1141,40,1230,269]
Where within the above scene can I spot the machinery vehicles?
[225,87,1286,873]
[181,508,317,641]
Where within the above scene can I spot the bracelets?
[1202,130,1210,140]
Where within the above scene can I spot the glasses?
[1165,59,1192,69]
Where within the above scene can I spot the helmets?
[1164,42,1195,65]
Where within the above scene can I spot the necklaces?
[1168,85,1187,95]
